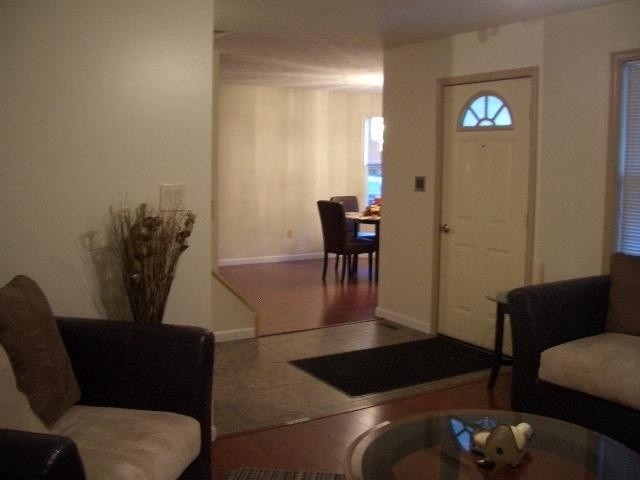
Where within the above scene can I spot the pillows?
[0,275,84,421]
[0,344,55,435]
[604,252,640,336]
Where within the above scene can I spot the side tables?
[484,289,513,390]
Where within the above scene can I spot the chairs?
[507,274,640,455]
[316,196,382,283]
[0,317,215,480]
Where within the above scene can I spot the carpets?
[224,466,345,480]
[288,336,509,399]
